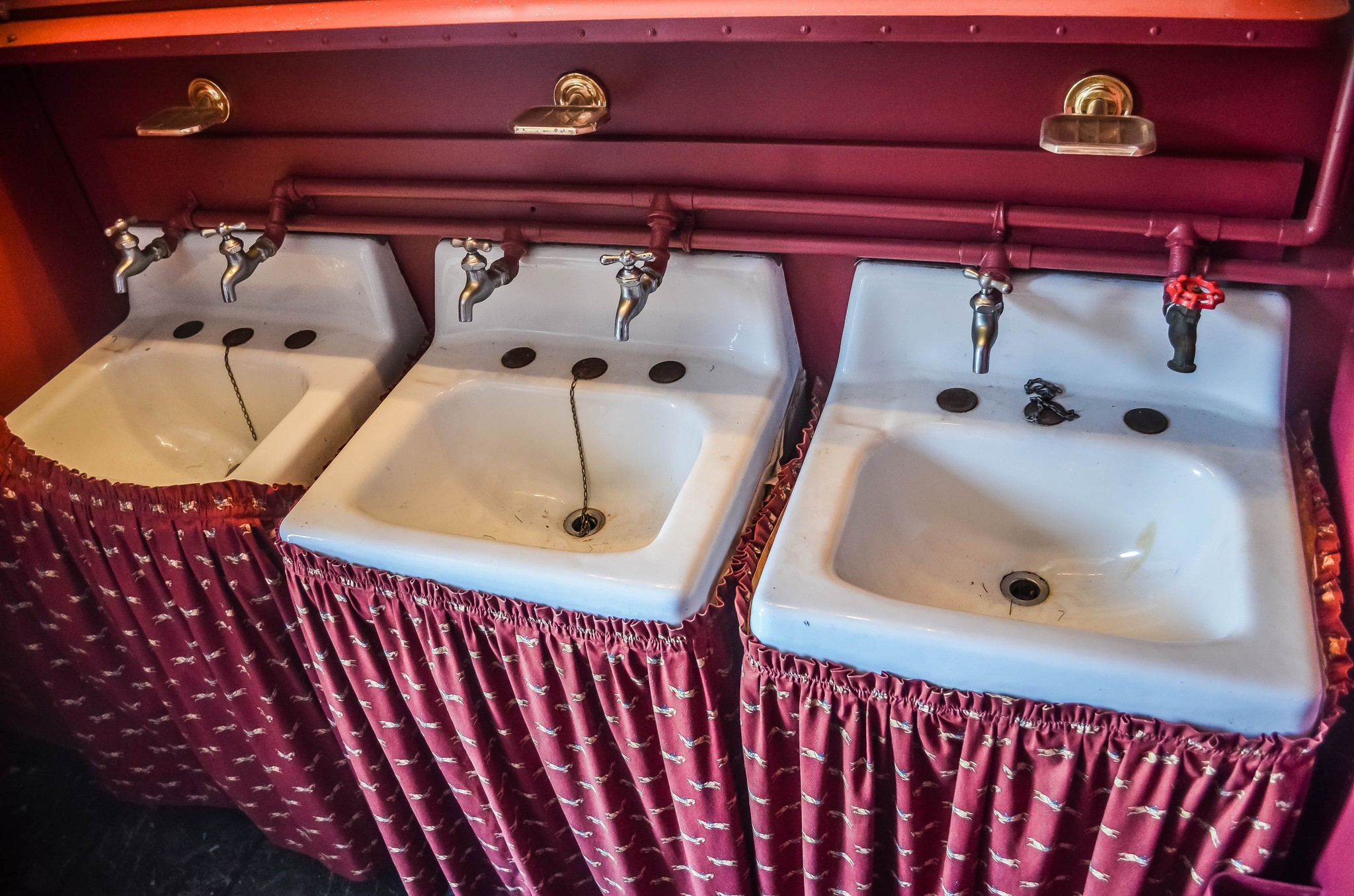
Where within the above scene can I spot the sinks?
[1,218,429,484]
[749,247,1328,743]
[276,228,805,633]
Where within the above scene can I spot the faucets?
[1161,275,1223,379]
[198,219,271,306]
[104,210,165,297]
[446,233,507,324]
[598,249,661,344]
[962,266,1013,369]
